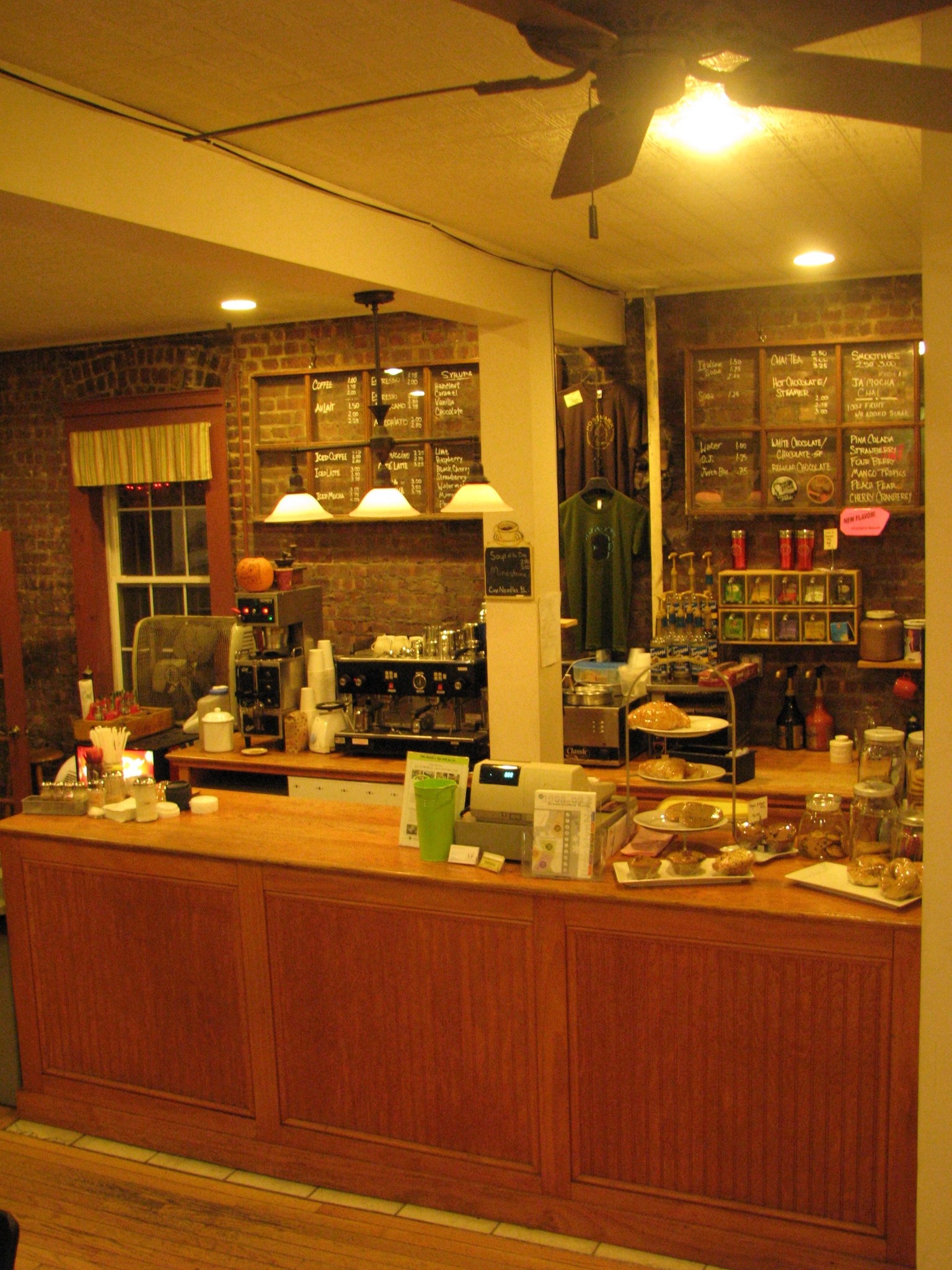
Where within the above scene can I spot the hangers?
[581,371,613,384]
[580,457,615,495]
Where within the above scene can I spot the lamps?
[263,289,513,523]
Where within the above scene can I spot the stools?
[5,749,64,817]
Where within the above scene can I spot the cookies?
[907,768,924,807]
[798,824,849,860]
[854,841,891,859]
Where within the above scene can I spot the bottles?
[798,715,924,861]
[197,685,230,748]
[309,702,347,754]
[858,610,904,662]
[804,677,834,751]
[774,678,804,750]
[480,602,486,624]
[40,769,191,823]
[903,619,926,663]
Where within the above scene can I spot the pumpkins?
[236,557,274,592]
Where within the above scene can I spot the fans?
[445,0,952,197]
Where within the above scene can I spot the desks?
[122,731,197,752]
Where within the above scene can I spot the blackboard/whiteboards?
[484,546,532,598]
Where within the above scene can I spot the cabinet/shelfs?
[249,359,482,525]
[680,332,925,515]
[719,568,863,645]
[164,761,402,809]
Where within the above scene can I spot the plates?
[241,747,268,755]
[720,845,798,863]
[784,862,922,909]
[612,858,754,885]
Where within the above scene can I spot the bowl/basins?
[564,660,628,706]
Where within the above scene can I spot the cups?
[275,568,294,590]
[731,529,748,569]
[779,529,815,570]
[298,639,335,729]
[414,778,457,862]
[370,620,479,663]
[892,672,919,702]
[201,707,235,752]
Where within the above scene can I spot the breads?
[627,700,723,828]
[846,854,924,900]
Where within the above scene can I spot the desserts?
[627,849,754,879]
[734,820,797,853]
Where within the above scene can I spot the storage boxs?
[667,744,757,784]
[74,707,172,742]
